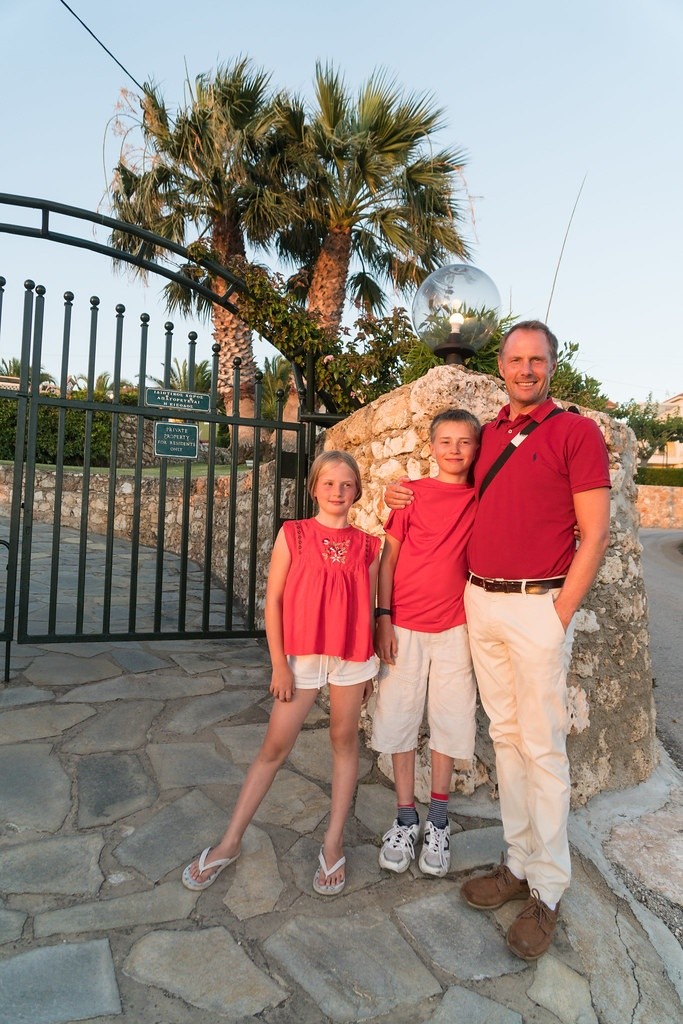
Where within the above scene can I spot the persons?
[383,320,614,960]
[181,450,382,896]
[366,408,581,878]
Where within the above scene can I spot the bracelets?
[374,607,391,618]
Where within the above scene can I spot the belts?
[465,570,566,595]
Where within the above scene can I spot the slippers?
[313,842,345,896]
[181,846,242,891]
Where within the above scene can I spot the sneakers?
[378,810,421,874]
[506,888,560,961]
[419,814,451,880]
[460,851,529,910]
